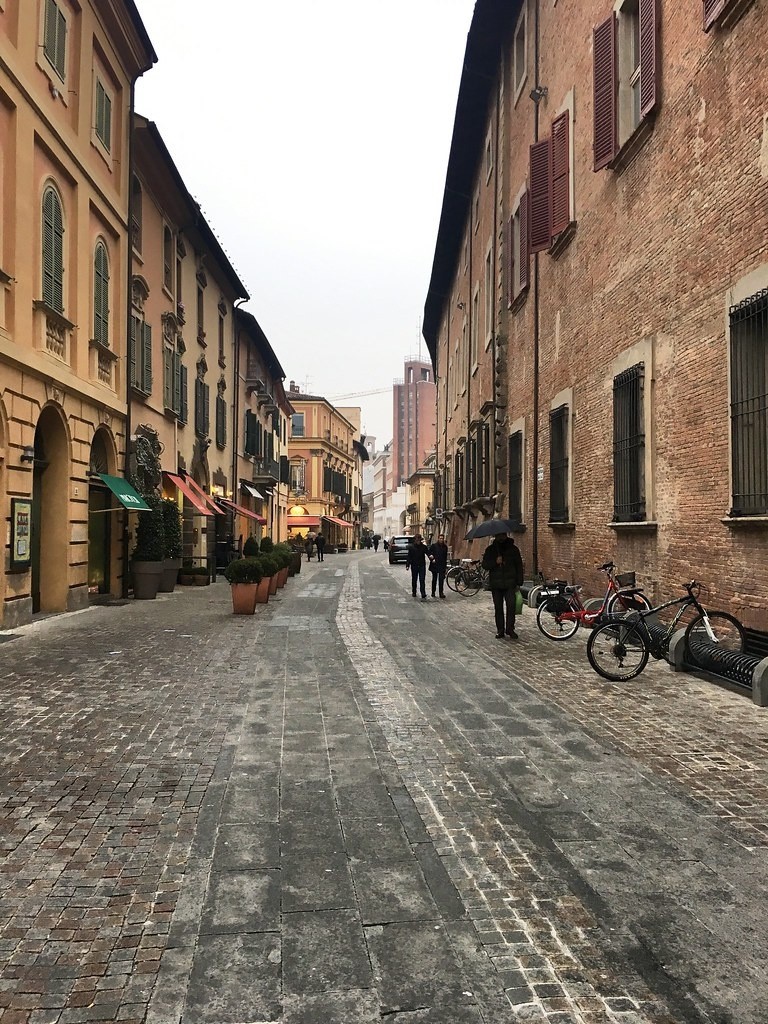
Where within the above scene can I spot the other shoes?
[440,593,446,598]
[317,560,320,562]
[431,592,435,597]
[321,559,324,561]
[412,592,416,597]
[308,559,310,561]
[421,592,426,598]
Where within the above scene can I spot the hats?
[318,532,322,535]
[413,533,424,541]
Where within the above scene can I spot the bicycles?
[534,561,652,641]
[587,578,747,682]
[446,558,489,598]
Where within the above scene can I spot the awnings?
[86,472,153,513]
[322,516,354,527]
[161,472,227,517]
[286,515,320,526]
[221,498,269,525]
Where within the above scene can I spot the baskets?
[615,571,635,587]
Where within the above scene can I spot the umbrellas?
[463,519,526,568]
[305,531,317,539]
[372,535,381,541]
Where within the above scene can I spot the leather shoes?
[495,632,504,638]
[505,627,518,639]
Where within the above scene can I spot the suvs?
[387,535,414,564]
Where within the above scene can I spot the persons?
[428,534,449,598]
[314,532,326,562]
[405,533,436,598]
[360,536,390,551]
[305,537,315,562]
[482,532,524,639]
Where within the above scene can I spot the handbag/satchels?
[516,585,523,614]
